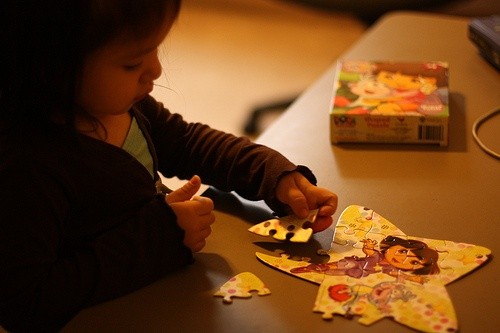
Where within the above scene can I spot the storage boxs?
[329,57,449,147]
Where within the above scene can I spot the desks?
[61,12,500,333]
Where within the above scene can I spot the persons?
[0,0,339,333]
